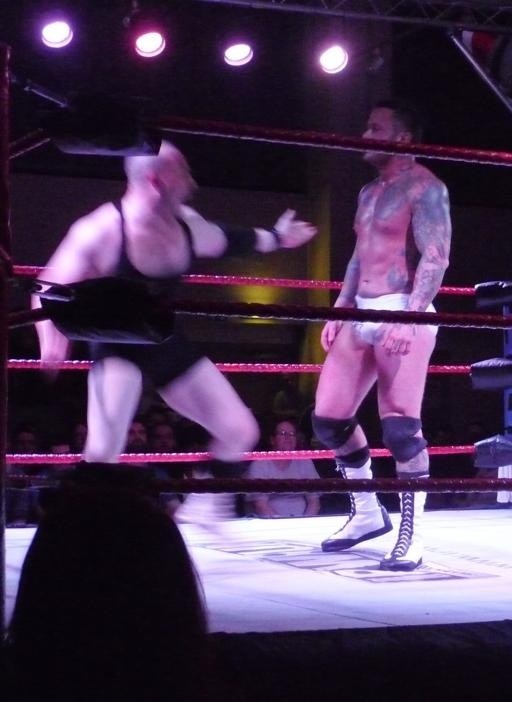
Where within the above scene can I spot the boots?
[379,474,431,572]
[321,455,393,552]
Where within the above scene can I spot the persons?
[305,92,456,573]
[272,373,304,422]
[32,139,321,555]
[243,420,320,521]
[0,472,214,702]
[1,412,180,527]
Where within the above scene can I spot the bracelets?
[265,225,284,251]
